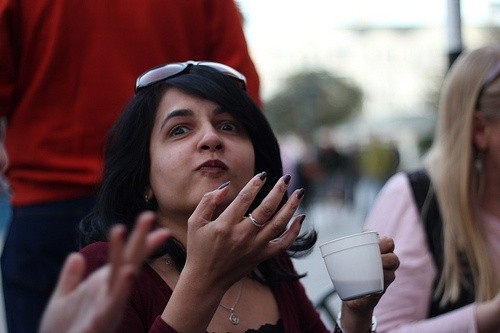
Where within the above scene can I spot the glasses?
[135,60,248,94]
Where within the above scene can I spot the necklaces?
[159,252,245,325]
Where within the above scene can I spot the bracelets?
[334,309,377,333]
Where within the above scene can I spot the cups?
[319,231,384,301]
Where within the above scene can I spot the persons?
[363,43,500,333]
[77,61,399,333]
[0,1,262,333]
[38,212,173,333]
[276,119,401,217]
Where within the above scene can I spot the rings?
[246,214,261,229]
